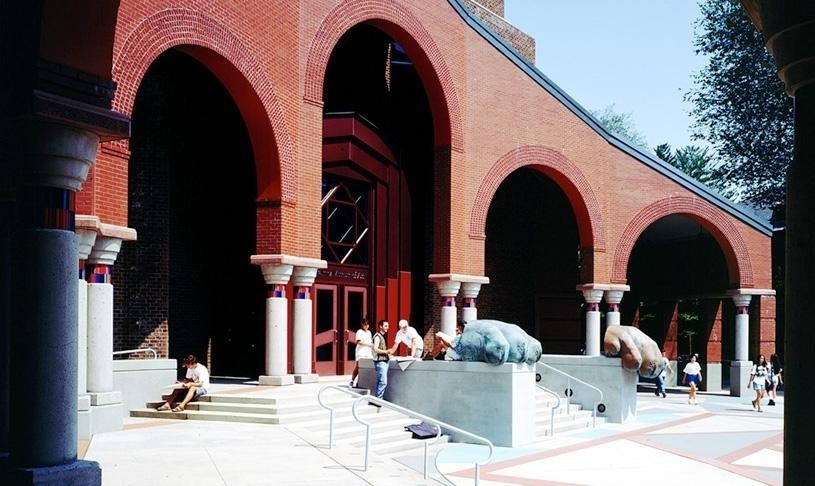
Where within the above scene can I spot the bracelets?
[670,368,671,371]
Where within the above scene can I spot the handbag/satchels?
[764,380,774,393]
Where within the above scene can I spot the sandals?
[157,405,185,412]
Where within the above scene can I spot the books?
[436,330,454,342]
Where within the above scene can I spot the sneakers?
[751,399,776,412]
[688,398,699,405]
[654,389,667,398]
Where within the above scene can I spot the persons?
[747,354,771,412]
[388,319,424,357]
[369,319,391,407]
[423,319,467,360]
[766,353,784,405]
[349,319,374,387]
[157,354,209,412]
[681,354,703,405]
[654,349,674,398]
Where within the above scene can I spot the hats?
[399,320,408,327]
